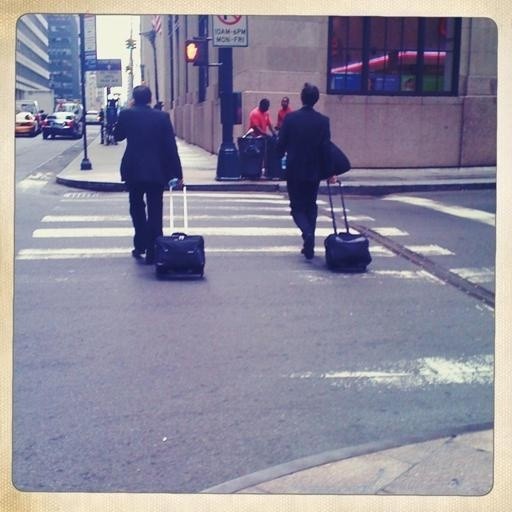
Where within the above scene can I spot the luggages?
[324,180,371,271]
[155,184,204,279]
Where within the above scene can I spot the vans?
[54,103,84,120]
[15,100,41,127]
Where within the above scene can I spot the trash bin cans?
[215,137,288,181]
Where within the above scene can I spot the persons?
[113,85,184,256]
[274,96,293,136]
[98,108,106,124]
[247,97,277,140]
[278,82,338,260]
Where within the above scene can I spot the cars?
[84,110,100,123]
[15,110,38,137]
[42,111,84,140]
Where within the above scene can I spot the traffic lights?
[107,87,110,94]
[183,34,207,68]
[125,38,131,48]
[132,40,137,49]
